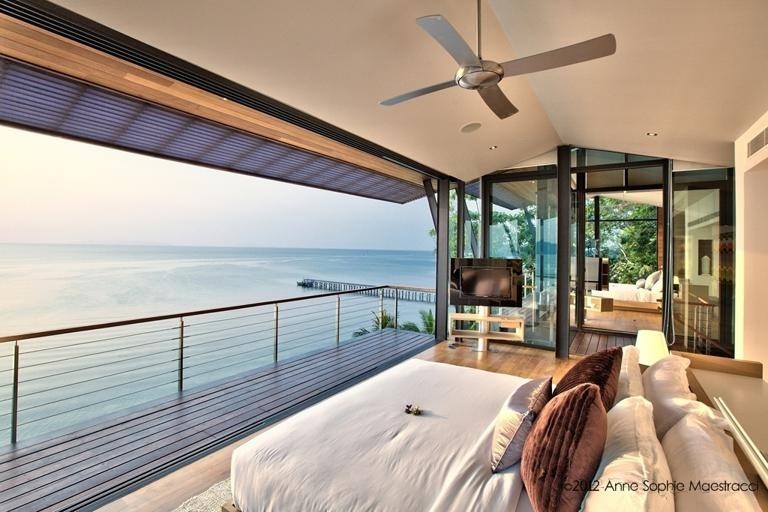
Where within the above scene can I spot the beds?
[222,358,557,511]
[591,283,660,313]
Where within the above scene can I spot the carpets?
[172,477,232,511]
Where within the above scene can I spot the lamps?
[636,330,669,366]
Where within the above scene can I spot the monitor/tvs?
[450,258,523,307]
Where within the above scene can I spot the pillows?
[636,271,663,291]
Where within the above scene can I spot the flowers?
[405,405,423,415]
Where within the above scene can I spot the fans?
[381,0,616,120]
